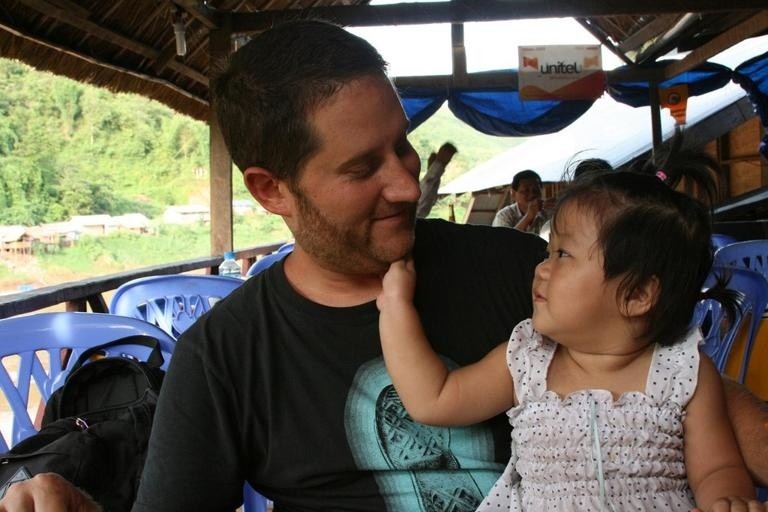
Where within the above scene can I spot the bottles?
[218,251,242,280]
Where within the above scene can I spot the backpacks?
[0,335,166,512]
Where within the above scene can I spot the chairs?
[689,232,767,387]
[0,240,297,511]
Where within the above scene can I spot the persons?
[376,124,768,512]
[0,19,768,512]
[572,157,612,182]
[492,170,555,235]
